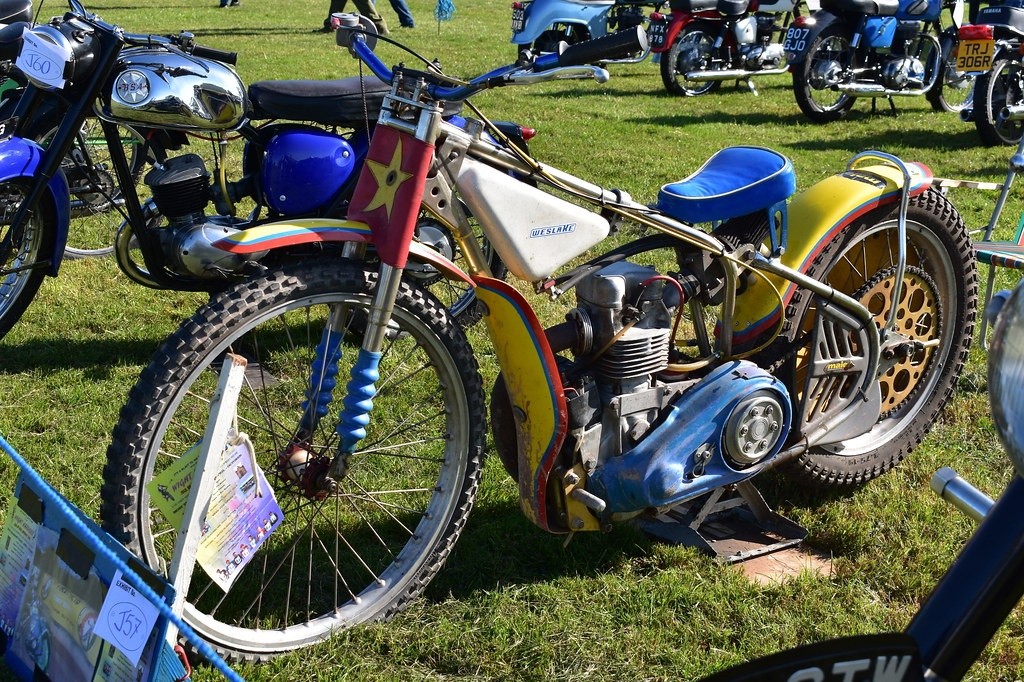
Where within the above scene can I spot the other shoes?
[312,27,334,33]
[399,22,414,28]
[230,1,239,6]
[219,4,228,8]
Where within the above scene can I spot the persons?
[220,0,239,8]
[371,0,415,27]
[313,0,390,34]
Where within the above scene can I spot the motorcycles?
[0,2,1024,682]
